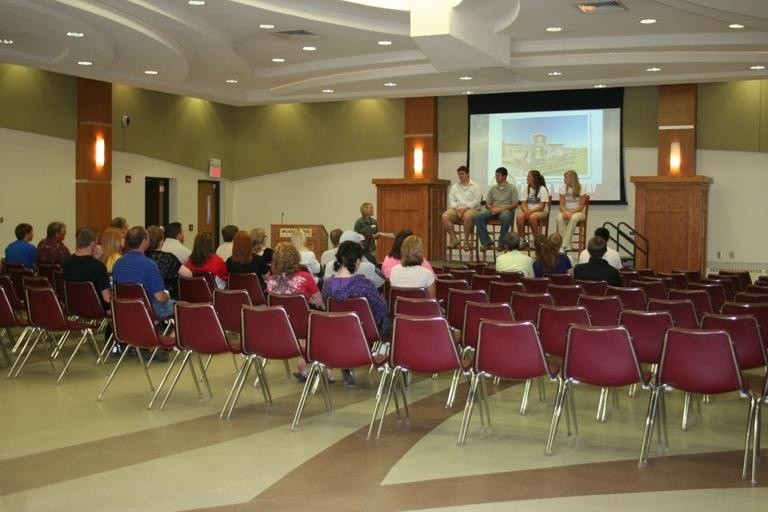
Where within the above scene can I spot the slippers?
[292,373,306,382]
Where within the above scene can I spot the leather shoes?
[341,368,354,385]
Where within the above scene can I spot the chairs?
[447,195,590,266]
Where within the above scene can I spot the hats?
[338,230,365,245]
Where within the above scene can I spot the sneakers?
[463,242,469,251]
[447,240,459,248]
[480,241,494,251]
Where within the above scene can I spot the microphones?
[281,213,284,224]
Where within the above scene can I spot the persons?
[440,164,483,252]
[471,166,520,252]
[514,169,550,251]
[354,202,379,255]
[194,224,444,380]
[496,226,623,287]
[553,168,587,256]
[6,217,192,360]
